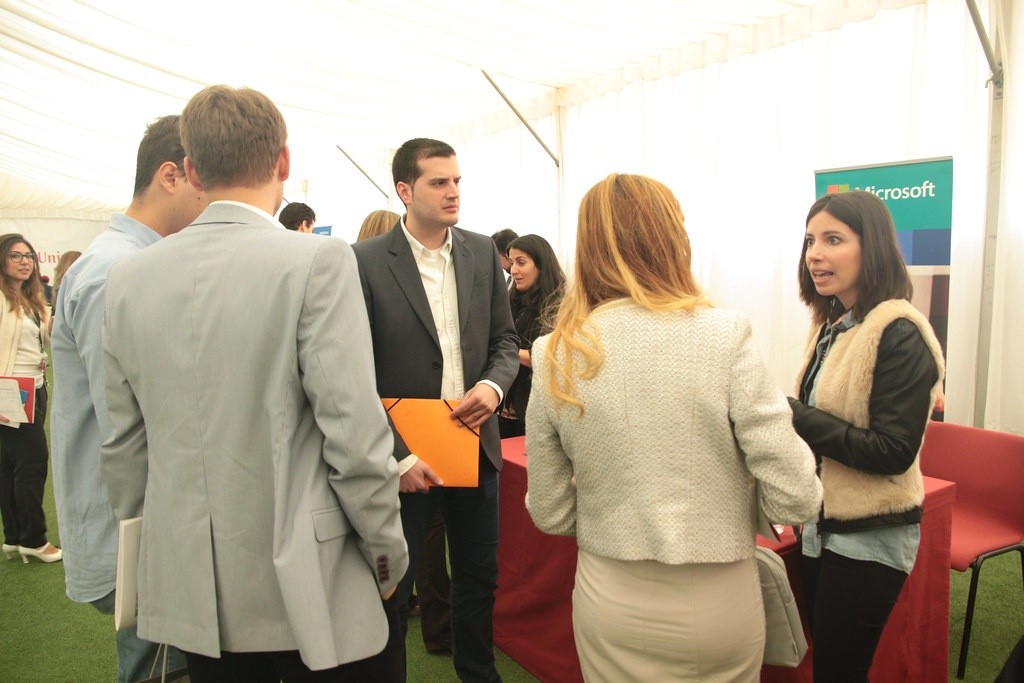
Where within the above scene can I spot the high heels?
[18,542,62,564]
[2,543,18,560]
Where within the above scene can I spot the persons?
[355,210,402,243]
[0,233,63,563]
[50,112,194,683]
[351,136,521,683]
[526,172,823,683]
[96,84,412,683]
[486,226,568,440]
[51,250,82,317]
[40,274,52,304]
[783,189,946,683]
[279,202,316,235]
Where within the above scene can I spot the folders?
[380,399,480,488]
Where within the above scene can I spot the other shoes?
[407,594,421,615]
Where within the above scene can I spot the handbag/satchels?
[754,545,808,667]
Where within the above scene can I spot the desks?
[494,432,956,683]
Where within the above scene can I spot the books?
[113,516,143,632]
[380,396,479,488]
[0,376,35,428]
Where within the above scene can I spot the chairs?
[918,422,1024,679]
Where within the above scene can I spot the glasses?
[6,252,34,263]
